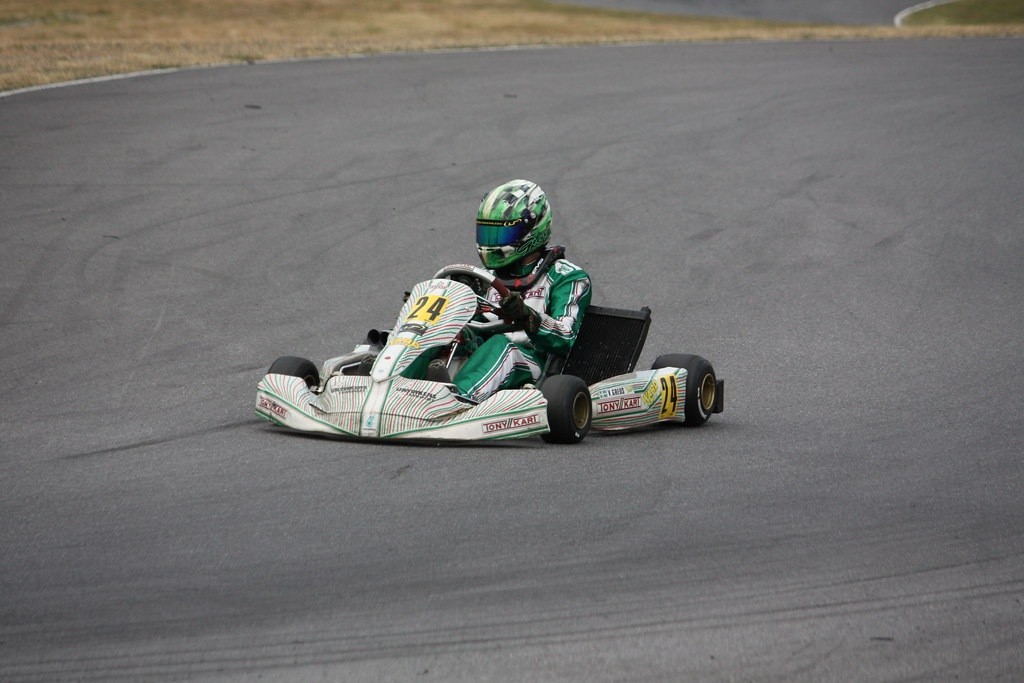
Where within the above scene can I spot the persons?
[358,179,593,406]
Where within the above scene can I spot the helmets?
[475,178,551,268]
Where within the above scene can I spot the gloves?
[499,292,529,324]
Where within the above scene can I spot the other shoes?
[360,354,376,375]
[427,359,451,383]
